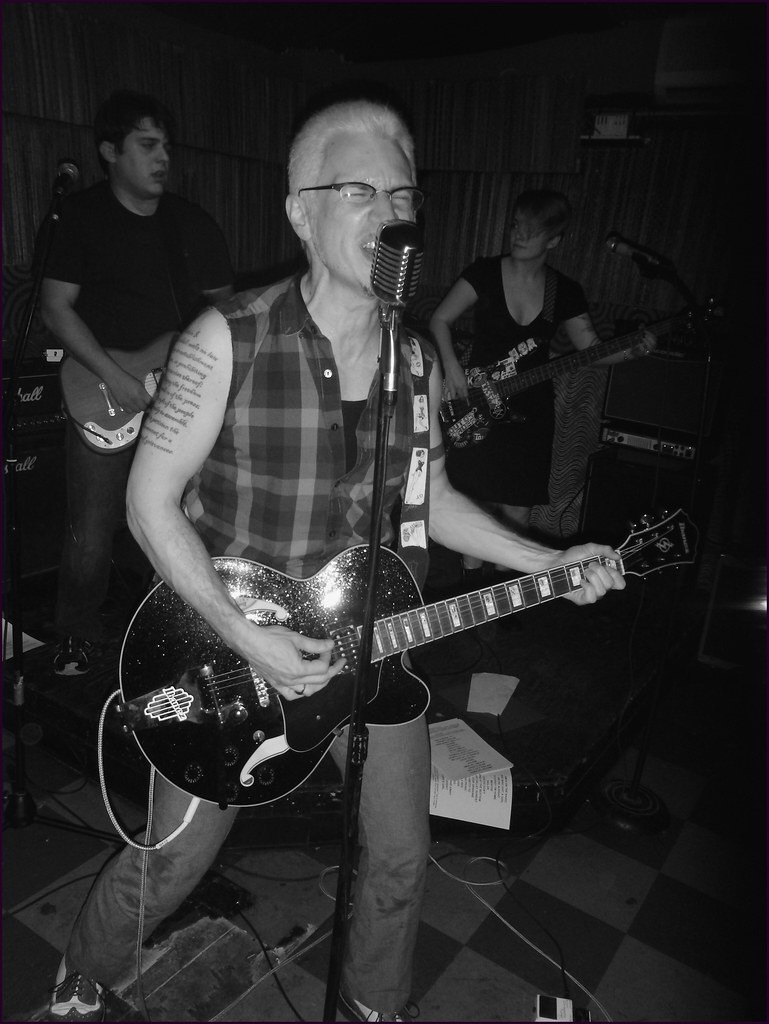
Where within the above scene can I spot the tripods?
[0,221,129,847]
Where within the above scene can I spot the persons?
[43,87,237,680]
[428,183,660,592]
[44,98,628,1023]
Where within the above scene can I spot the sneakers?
[53,634,97,674]
[337,989,404,1022]
[48,949,106,1022]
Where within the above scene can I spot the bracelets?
[623,349,628,360]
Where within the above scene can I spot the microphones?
[49,162,79,223]
[368,219,427,419]
[607,235,668,271]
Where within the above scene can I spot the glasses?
[298,182,429,213]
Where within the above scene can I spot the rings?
[295,684,305,695]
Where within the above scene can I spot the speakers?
[0,432,68,588]
[578,443,717,606]
[602,340,730,444]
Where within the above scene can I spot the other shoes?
[495,614,522,633]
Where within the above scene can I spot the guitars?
[116,509,703,811]
[58,330,175,457]
[436,290,719,455]
